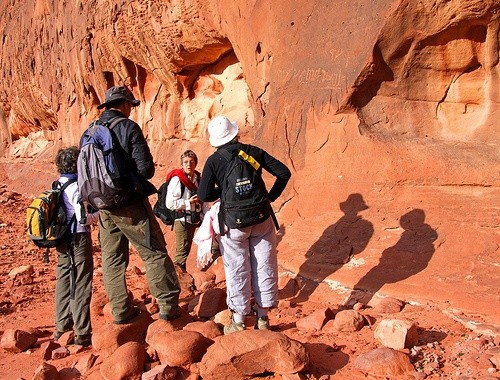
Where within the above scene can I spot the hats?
[208,116,239,147]
[95,85,141,109]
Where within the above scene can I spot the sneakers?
[158,305,183,321]
[74,332,92,345]
[254,316,270,330]
[224,316,245,336]
[113,306,141,324]
[53,330,64,341]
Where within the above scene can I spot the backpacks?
[152,176,187,226]
[217,143,281,236]
[26,180,76,248]
[76,115,128,213]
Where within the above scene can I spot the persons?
[195,116,291,337]
[53,145,101,345]
[166,150,203,271]
[79,83,188,324]
